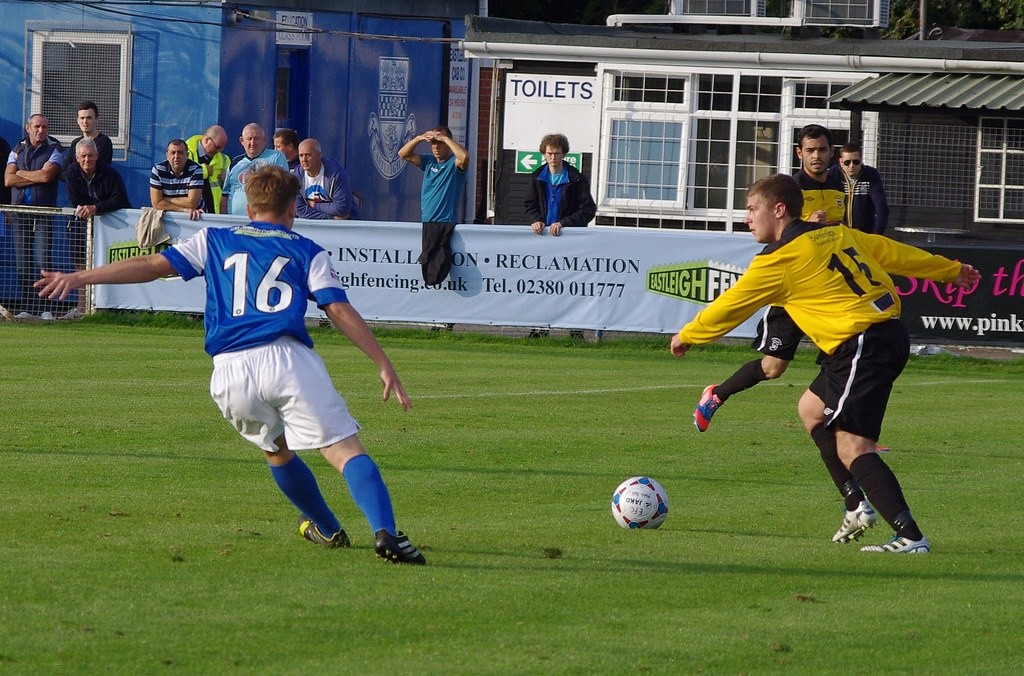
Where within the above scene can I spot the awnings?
[826,75,1024,109]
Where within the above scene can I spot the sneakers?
[40,311,55,321]
[874,444,892,454]
[859,531,931,554]
[693,383,725,432]
[372,528,427,565]
[63,308,85,320]
[297,513,350,549]
[832,500,878,543]
[14,311,33,319]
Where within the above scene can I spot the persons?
[694,124,847,430]
[219,124,289,216]
[0,137,12,235]
[831,142,889,235]
[293,139,359,327]
[67,138,132,319]
[395,126,469,330]
[185,124,230,214]
[524,134,596,341]
[149,139,208,319]
[671,175,982,554]
[3,115,62,320]
[273,130,299,168]
[57,101,112,318]
[31,166,427,564]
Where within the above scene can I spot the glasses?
[210,138,224,152]
[842,159,862,167]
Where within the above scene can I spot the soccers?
[611,475,670,530]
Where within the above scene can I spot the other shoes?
[569,330,586,347]
[431,323,455,333]
[317,319,332,332]
[528,329,549,339]
